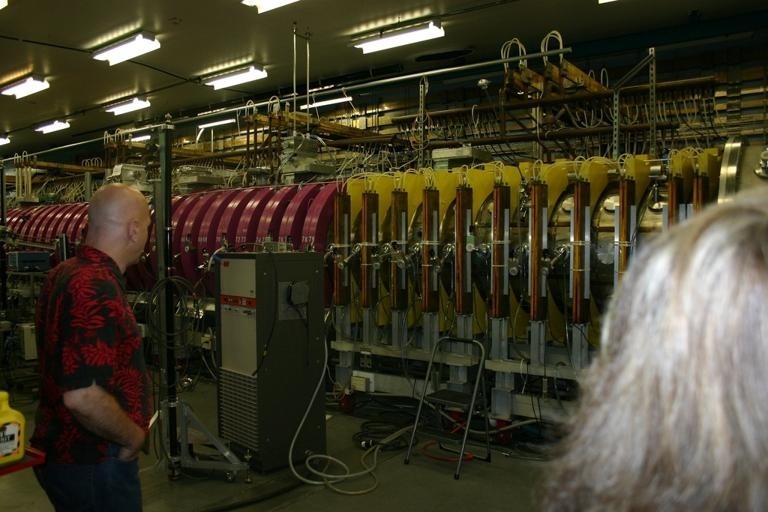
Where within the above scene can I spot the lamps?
[353,18,446,55]
[34,118,70,134]
[92,29,162,66]
[104,95,150,117]
[0,135,11,147]
[0,73,50,100]
[201,63,263,92]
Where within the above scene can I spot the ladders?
[404,337,492,480]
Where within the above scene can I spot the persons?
[30,183,155,512]
[533,186,768,512]
